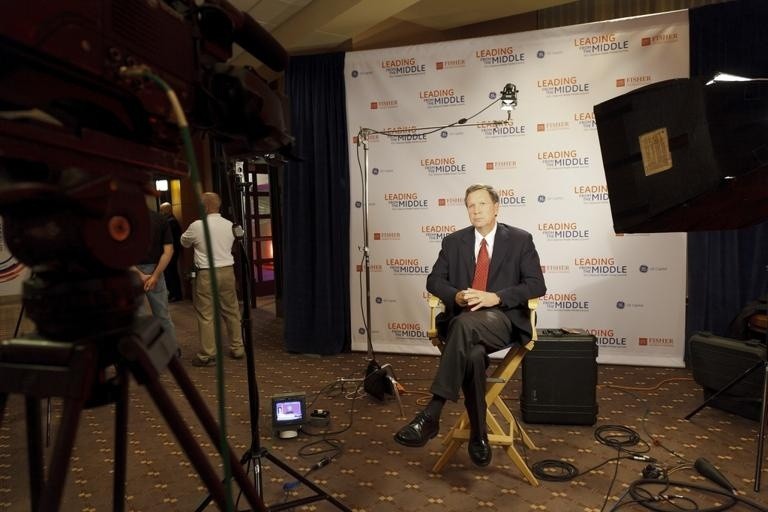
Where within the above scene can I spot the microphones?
[694,458,738,494]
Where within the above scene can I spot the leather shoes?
[467,429,491,467]
[393,413,439,448]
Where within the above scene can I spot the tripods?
[195,169,355,512]
[685,357,768,492]
[0,275,262,510]
[331,132,406,419]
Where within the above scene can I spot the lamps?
[355,84,518,361]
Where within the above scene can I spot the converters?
[312,408,330,417]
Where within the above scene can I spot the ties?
[471,238,489,292]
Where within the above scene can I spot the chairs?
[424,295,540,487]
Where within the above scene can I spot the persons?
[176,191,248,367]
[157,201,186,304]
[121,204,184,359]
[391,185,548,469]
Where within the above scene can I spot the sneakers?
[191,357,216,367]
